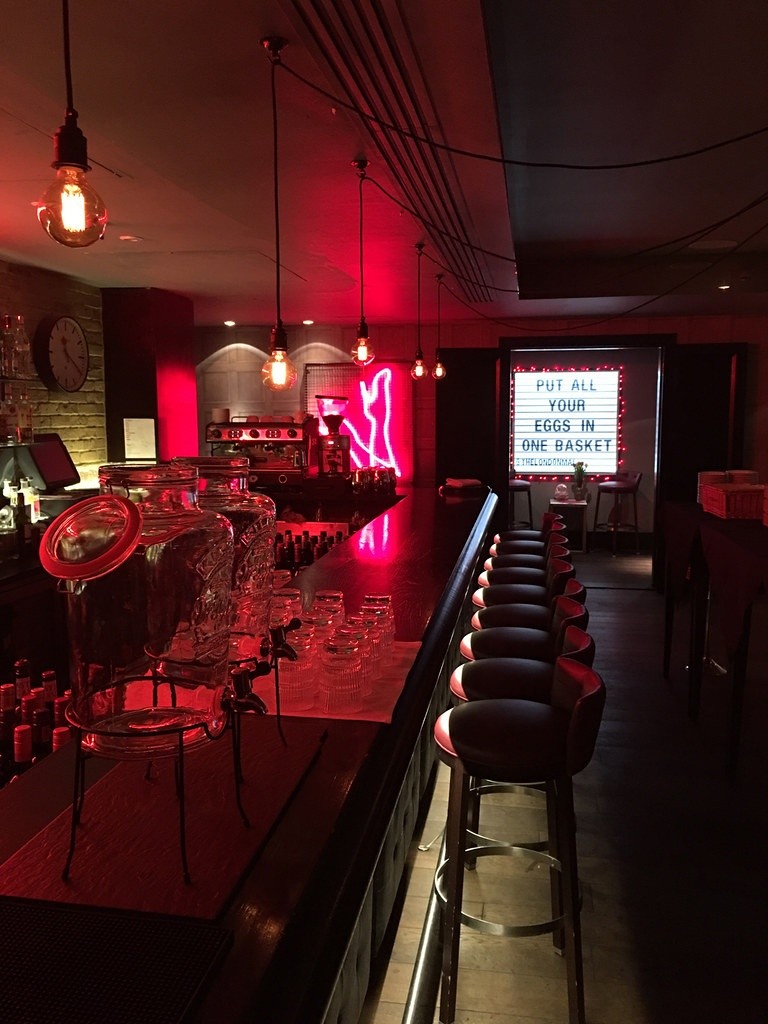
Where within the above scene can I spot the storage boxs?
[698,482,766,519]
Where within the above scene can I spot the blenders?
[315,395,350,478]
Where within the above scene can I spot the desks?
[660,502,768,773]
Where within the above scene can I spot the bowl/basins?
[212,409,229,423]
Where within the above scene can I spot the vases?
[572,483,586,501]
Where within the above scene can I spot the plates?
[696,470,758,503]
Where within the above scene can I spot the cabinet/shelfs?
[548,497,588,555]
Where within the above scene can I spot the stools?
[509,479,533,531]
[587,469,643,559]
[410,512,607,1024]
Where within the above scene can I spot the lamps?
[37,0,109,250]
[410,249,429,381]
[263,57,294,391]
[431,281,446,381]
[350,176,375,368]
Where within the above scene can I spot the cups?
[263,589,395,715]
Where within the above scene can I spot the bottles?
[145,456,277,678]
[15,383,33,443]
[0,383,18,443]
[293,450,301,467]
[0,476,40,528]
[301,451,307,467]
[0,658,124,793]
[62,462,234,754]
[276,531,351,569]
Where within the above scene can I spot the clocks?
[32,315,89,395]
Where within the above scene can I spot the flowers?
[572,461,588,488]
